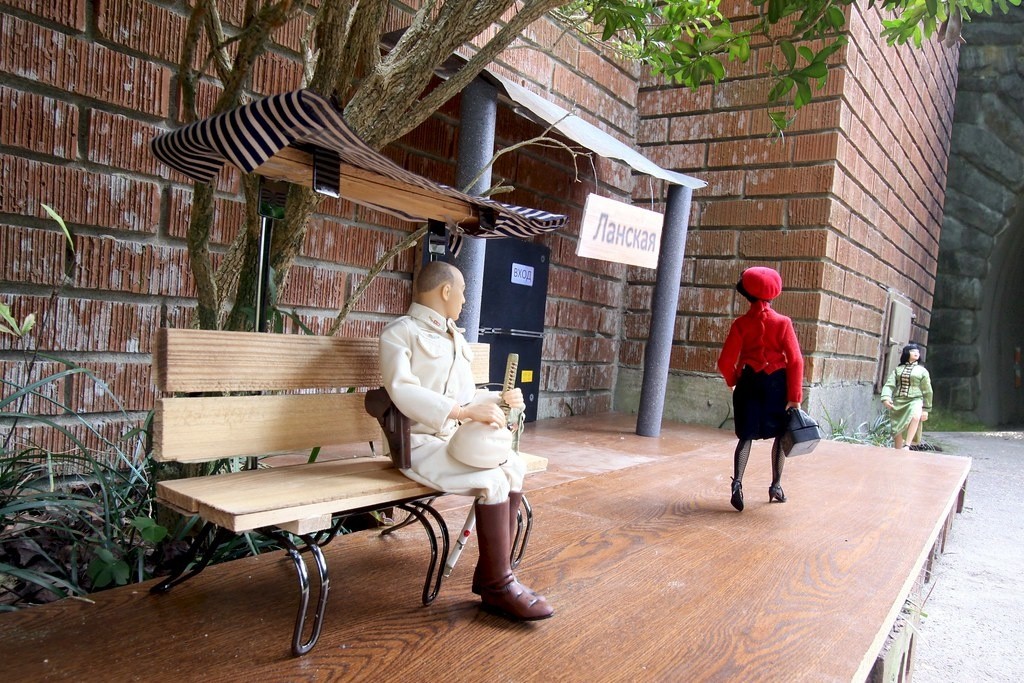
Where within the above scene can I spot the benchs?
[147,327,550,656]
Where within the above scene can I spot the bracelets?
[453,405,462,427]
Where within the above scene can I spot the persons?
[377,261,554,622]
[717,266,804,512]
[881,345,933,450]
